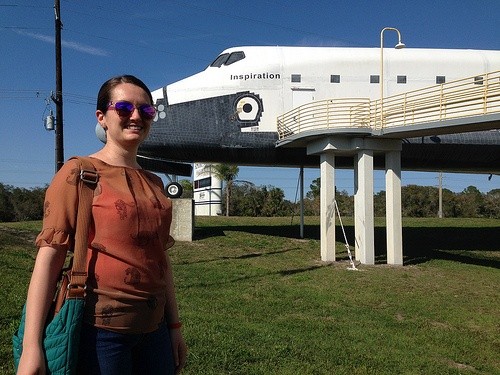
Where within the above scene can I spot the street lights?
[380,27,405,129]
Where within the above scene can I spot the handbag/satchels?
[10,278,86,375]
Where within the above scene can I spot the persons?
[16,75,190,374]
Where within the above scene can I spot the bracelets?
[167,322,182,330]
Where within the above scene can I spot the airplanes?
[96,44,500,200]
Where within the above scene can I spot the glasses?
[101,100,158,120]
